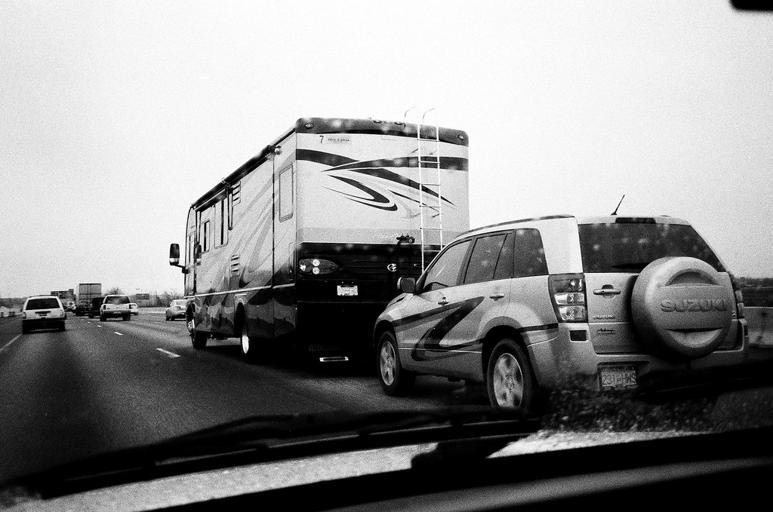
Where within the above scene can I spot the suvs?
[368,190,755,415]
[19,296,68,332]
[87,295,140,321]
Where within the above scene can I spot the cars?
[164,298,189,321]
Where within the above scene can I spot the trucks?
[50,282,102,319]
[167,114,476,370]
